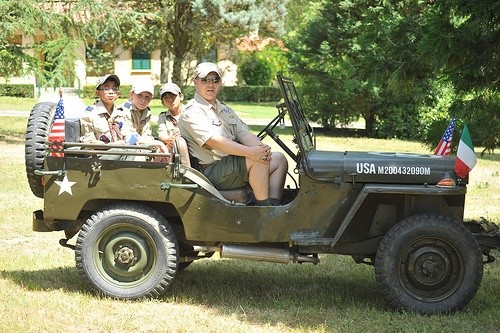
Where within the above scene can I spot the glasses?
[200,77,221,83]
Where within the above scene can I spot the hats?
[193,61,221,79]
[131,77,155,95]
[95,73,120,90]
[160,82,181,100]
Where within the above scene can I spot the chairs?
[175,137,252,202]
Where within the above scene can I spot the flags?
[434,117,455,155]
[455,125,477,178]
[48,98,65,157]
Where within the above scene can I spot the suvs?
[24,73,500,317]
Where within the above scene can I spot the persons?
[178,62,288,205]
[80,74,185,161]
